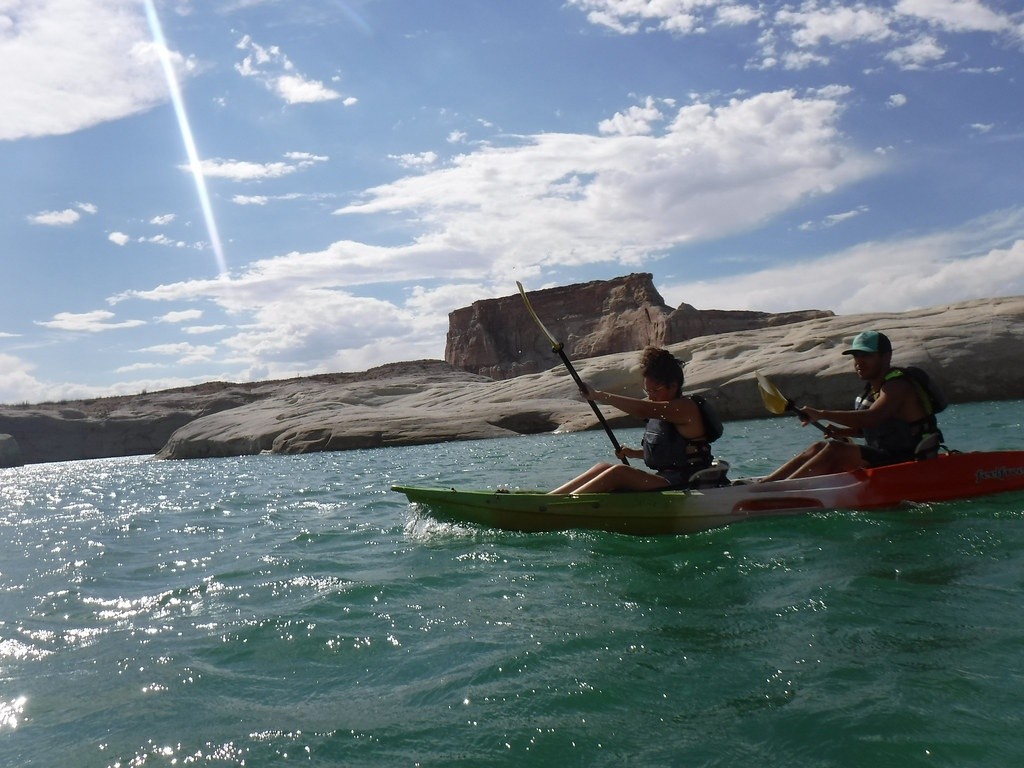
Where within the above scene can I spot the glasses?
[641,381,667,402]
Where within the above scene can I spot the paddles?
[755,370,853,443]
[516,280,631,466]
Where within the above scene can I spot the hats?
[842,331,893,355]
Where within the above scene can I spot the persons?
[546,346,712,494]
[758,332,936,482]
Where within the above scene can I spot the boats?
[390,451,1024,535]
